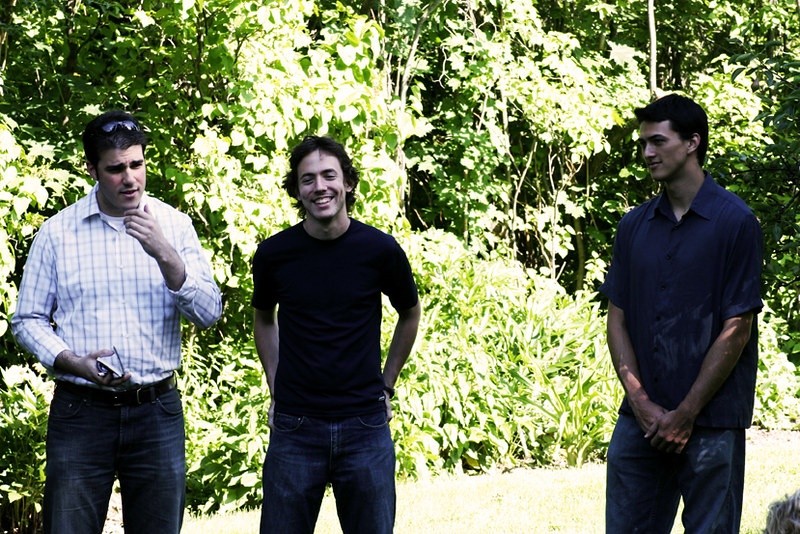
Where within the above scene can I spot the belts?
[56,376,176,405]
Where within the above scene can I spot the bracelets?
[383,386,395,399]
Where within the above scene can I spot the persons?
[8,111,224,534]
[600,93,769,534]
[245,135,421,534]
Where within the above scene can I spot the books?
[95,345,126,379]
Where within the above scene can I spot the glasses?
[94,120,137,137]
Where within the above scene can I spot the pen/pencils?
[98,372,106,376]
